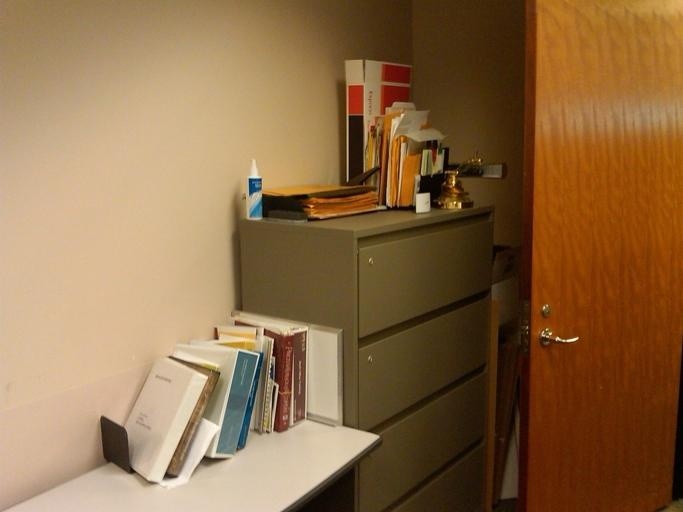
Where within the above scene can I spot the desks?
[0,420,383,512]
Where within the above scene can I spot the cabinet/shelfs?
[239,204,495,512]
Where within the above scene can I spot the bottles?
[244,157,264,221]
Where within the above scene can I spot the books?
[121,307,310,487]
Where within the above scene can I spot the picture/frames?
[231,311,343,426]
